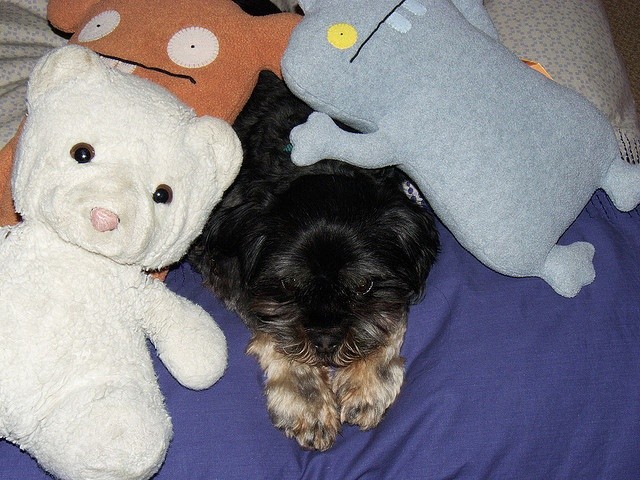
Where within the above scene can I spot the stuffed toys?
[280,1,640,298]
[0,43,243,480]
[0,1,305,284]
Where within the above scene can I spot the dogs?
[186,69,443,454]
